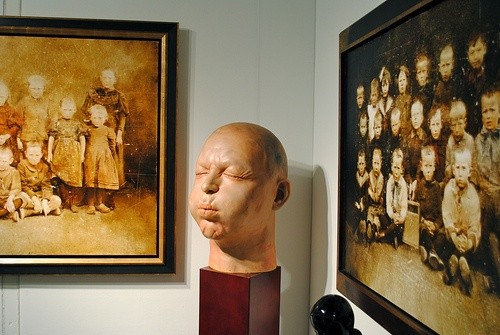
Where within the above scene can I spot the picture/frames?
[0,14,180,275]
[337,0,500,335]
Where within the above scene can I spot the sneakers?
[418,245,429,263]
[427,250,445,270]
[444,254,458,284]
[458,257,474,289]
[71,204,78,212]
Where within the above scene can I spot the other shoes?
[105,196,116,209]
[51,208,62,215]
[351,218,401,251]
[95,203,111,213]
[12,209,24,222]
[86,205,96,214]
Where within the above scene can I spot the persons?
[1,68,128,222]
[189,121,293,275]
[351,31,500,298]
